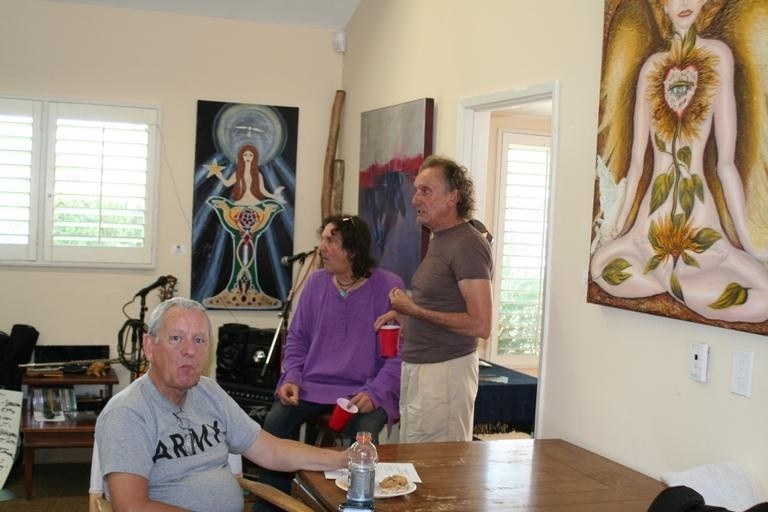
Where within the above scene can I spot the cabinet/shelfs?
[21,363,120,501]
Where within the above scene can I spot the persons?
[89,297,350,512]
[216,144,274,206]
[374,158,494,444]
[248,215,406,512]
[590,0,768,323]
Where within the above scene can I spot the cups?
[379,325,400,358]
[328,398,358,433]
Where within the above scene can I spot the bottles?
[347,432,377,510]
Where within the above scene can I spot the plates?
[335,471,417,499]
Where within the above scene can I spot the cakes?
[379,476,409,493]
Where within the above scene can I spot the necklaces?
[336,278,357,297]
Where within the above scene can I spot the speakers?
[216,323,282,478]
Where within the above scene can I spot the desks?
[286,436,673,512]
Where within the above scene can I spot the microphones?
[136,276,167,296]
[280,252,308,267]
